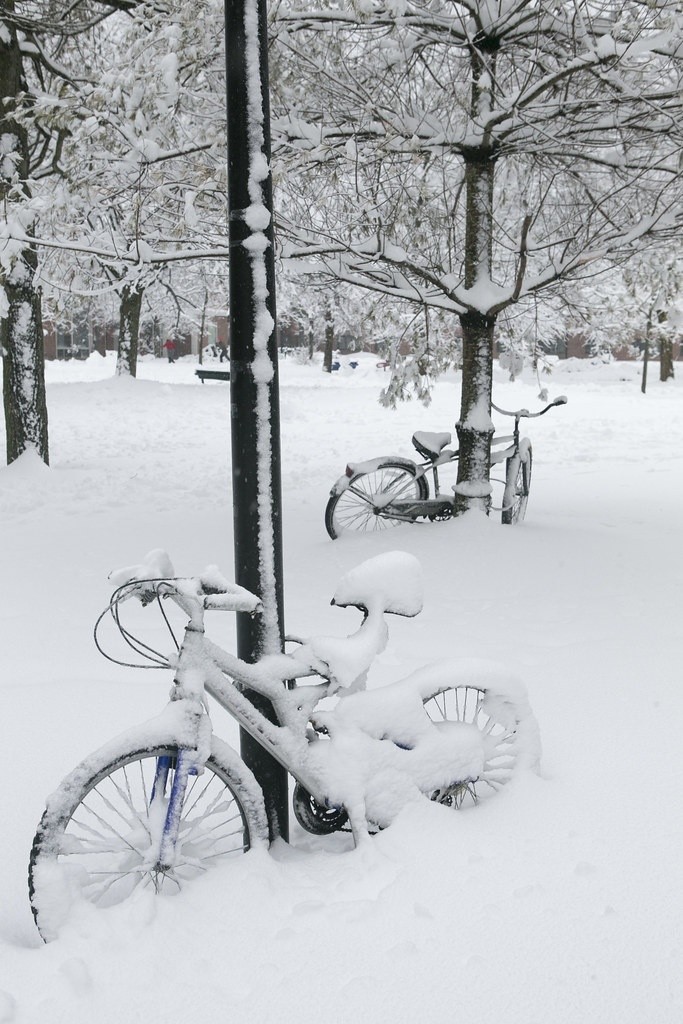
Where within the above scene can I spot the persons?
[217,341,230,362]
[161,339,175,364]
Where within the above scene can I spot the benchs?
[195,370,230,383]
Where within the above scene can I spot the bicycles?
[27,571,542,946]
[325,400,566,540]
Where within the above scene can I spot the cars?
[331,353,387,371]
